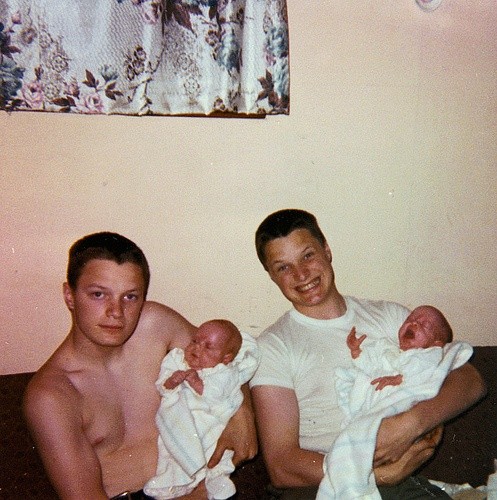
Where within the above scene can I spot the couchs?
[0,345,496,499]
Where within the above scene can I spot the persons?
[250,207,481,500]
[29,229,259,500]
[346,302,455,432]
[146,318,247,497]
